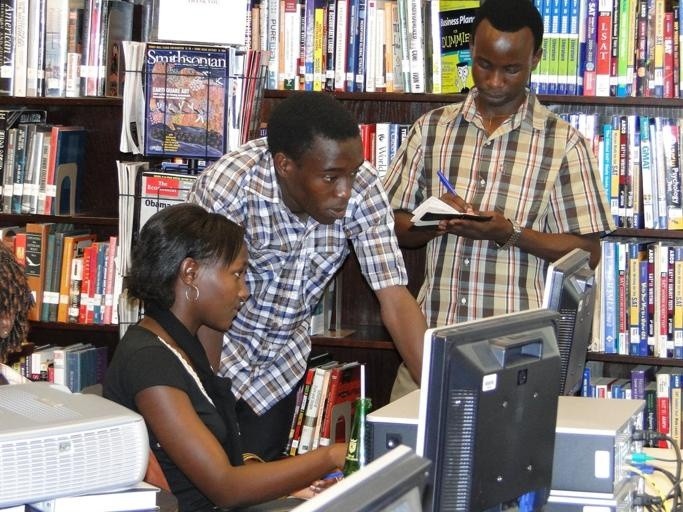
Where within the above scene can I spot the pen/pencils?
[325,472,345,480]
[436,170,458,195]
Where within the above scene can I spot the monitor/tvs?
[542,246,598,396]
[290,442,434,512]
[415,304,564,512]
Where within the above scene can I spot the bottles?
[342,399,374,478]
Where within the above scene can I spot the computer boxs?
[367,381,647,498]
[541,474,645,512]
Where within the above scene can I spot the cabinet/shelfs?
[0,0,683,456]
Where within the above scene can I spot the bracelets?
[241,452,265,463]
[496,217,521,250]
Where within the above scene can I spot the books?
[306,271,342,335]
[540,113,679,448]
[284,351,364,458]
[117,33,269,346]
[245,1,480,185]
[523,1,680,99]
[1,4,159,396]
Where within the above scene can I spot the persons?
[380,2,616,405]
[100,199,350,510]
[0,243,33,386]
[186,91,430,464]
